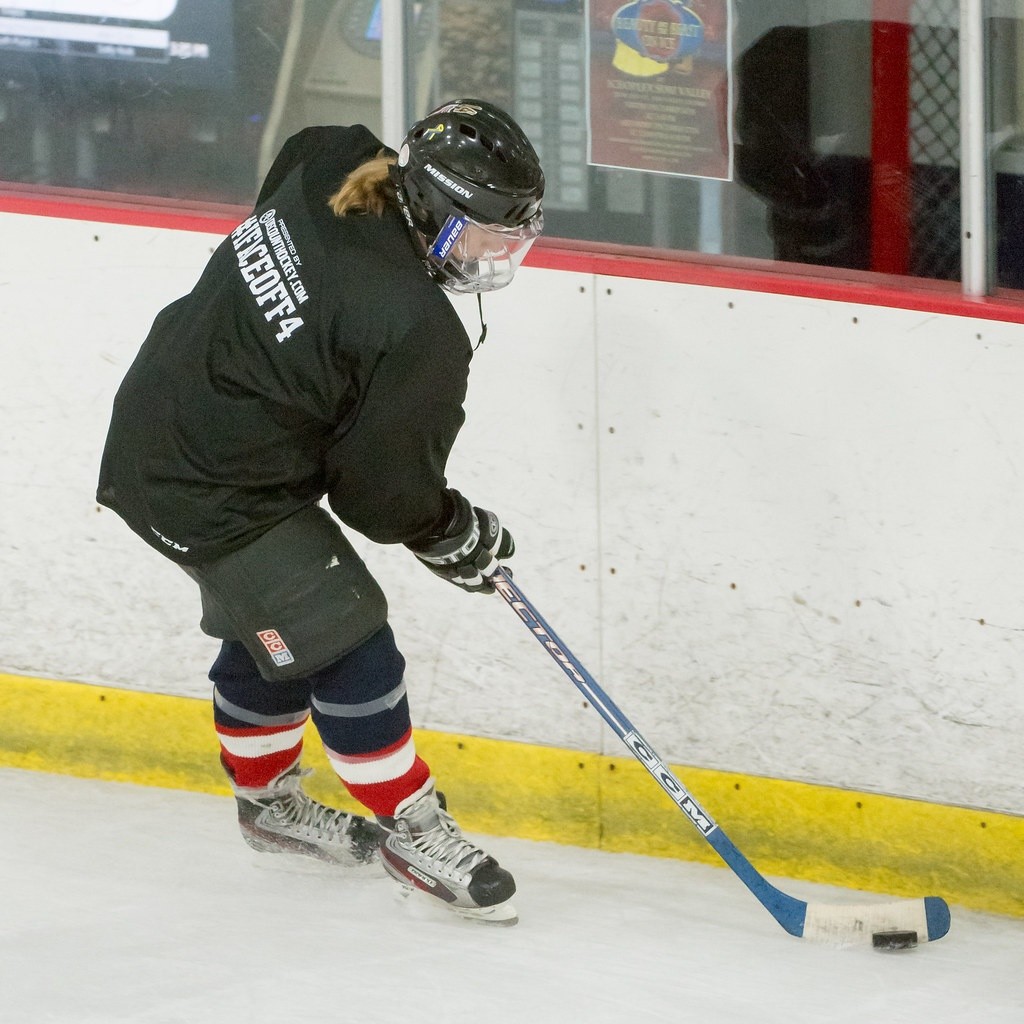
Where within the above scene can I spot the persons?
[94,97,547,925]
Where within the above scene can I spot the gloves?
[404,488,516,595]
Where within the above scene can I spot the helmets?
[398,97,545,241]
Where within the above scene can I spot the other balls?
[870,930,919,949]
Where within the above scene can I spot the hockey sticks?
[472,548,951,945]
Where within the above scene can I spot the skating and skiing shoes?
[379,787,519,928]
[221,756,391,876]
